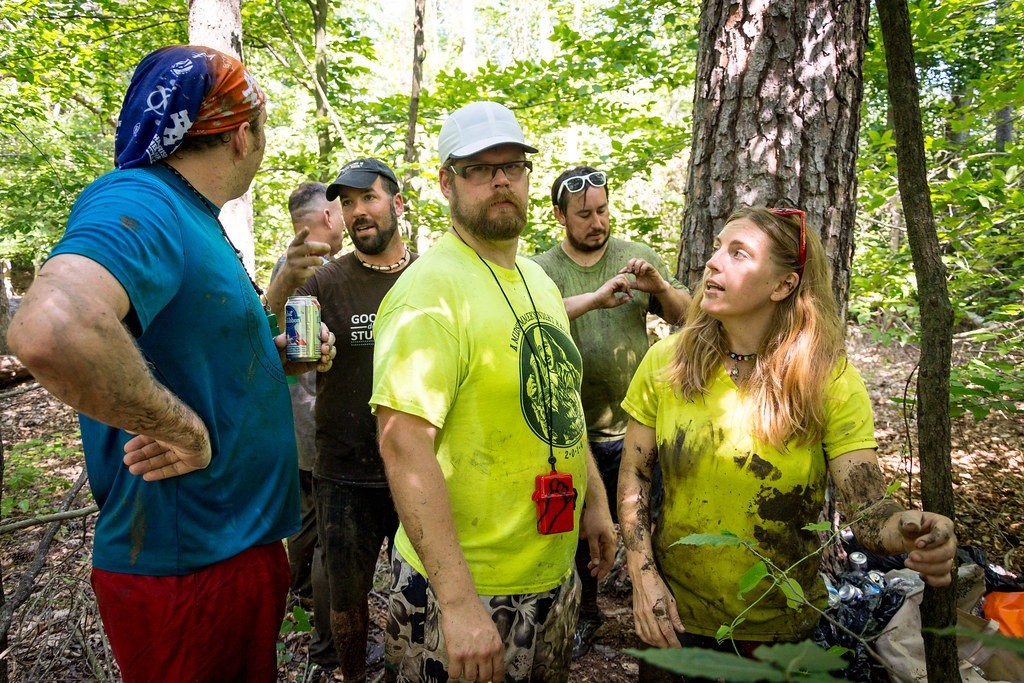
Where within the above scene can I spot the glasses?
[769,207,806,277]
[448,161,533,183]
[557,172,607,204]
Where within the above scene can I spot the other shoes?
[571,608,606,660]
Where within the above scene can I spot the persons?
[616,205,956,683]
[368,100,617,683]
[529,165,694,658]
[261,157,421,683]
[7,46,336,683]
[265,180,345,673]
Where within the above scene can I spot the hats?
[326,157,397,201]
[437,101,539,167]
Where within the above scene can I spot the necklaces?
[723,347,758,376]
[162,160,280,337]
[355,249,406,271]
[452,225,575,535]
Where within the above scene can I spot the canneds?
[821,528,886,624]
[285,295,322,362]
